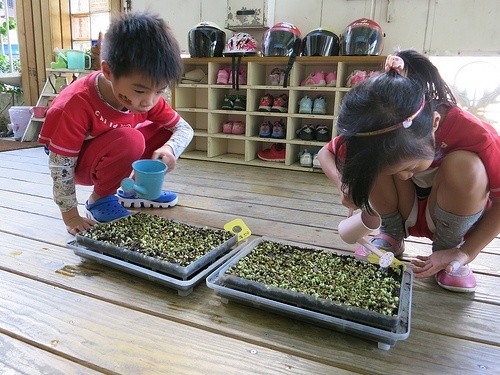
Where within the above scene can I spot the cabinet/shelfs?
[169,54,387,172]
[22,69,92,140]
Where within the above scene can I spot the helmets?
[302,26,342,56]
[342,18,385,56]
[262,22,302,57]
[188,20,226,57]
[222,32,259,56]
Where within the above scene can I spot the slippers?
[84,197,132,222]
[118,188,178,208]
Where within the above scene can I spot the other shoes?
[355,234,405,256]
[435,266,476,292]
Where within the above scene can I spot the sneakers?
[216,66,246,84]
[223,119,330,169]
[269,67,290,85]
[298,96,328,114]
[258,93,287,112]
[221,95,246,110]
[300,70,336,87]
[345,69,381,87]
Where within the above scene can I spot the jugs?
[50,45,92,70]
[48,72,77,94]
[120,158,168,201]
[29,106,50,118]
[338,208,395,268]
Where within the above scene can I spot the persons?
[37,9,195,236]
[318,50,500,291]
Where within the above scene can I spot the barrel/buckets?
[8,105,34,141]
